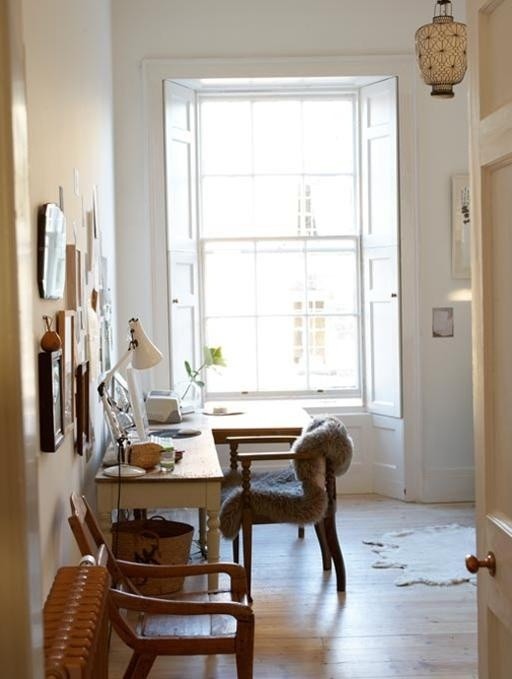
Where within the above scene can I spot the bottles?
[160,437,175,473]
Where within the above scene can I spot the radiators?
[42,554,113,679]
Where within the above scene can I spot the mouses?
[177,428,196,434]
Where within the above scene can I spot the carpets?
[362,521,477,589]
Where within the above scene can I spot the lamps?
[413,1,467,100]
[97,316,166,478]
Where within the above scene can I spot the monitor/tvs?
[126,362,150,442]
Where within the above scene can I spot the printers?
[145,390,195,424]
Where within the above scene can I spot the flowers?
[181,345,229,398]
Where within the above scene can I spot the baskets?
[112,512,194,595]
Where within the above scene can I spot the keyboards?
[150,434,174,452]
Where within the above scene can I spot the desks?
[94,399,315,594]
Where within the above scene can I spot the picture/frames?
[39,243,94,462]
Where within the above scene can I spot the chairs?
[226,415,346,605]
[67,491,255,678]
